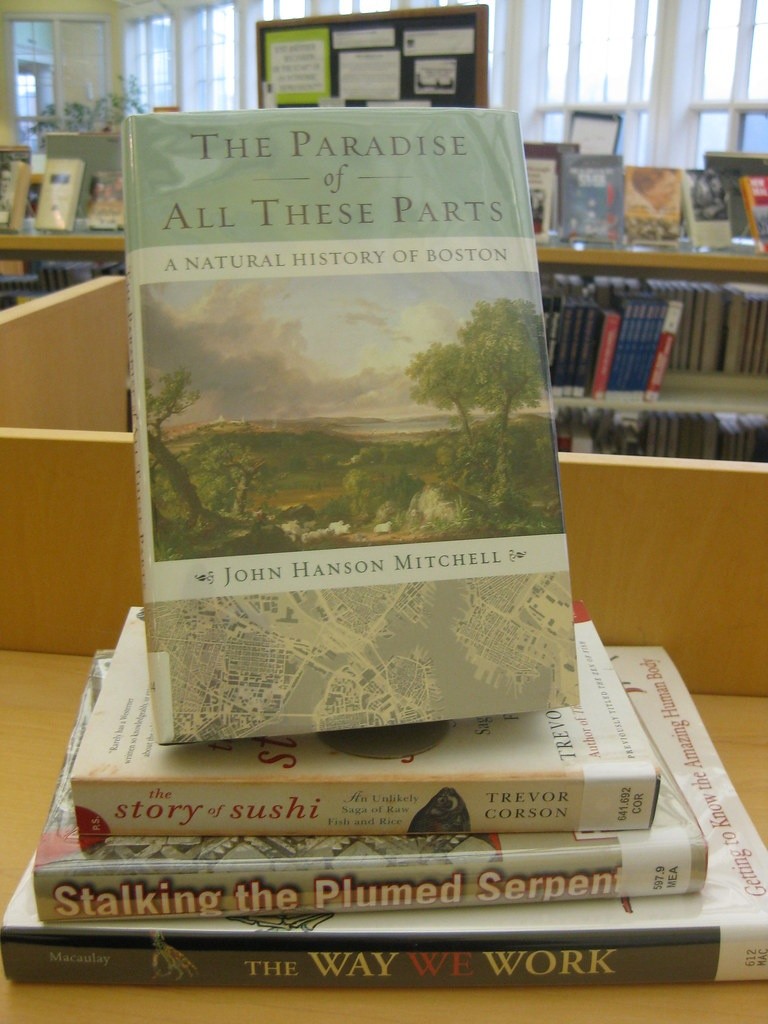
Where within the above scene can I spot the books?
[543,273,768,463]
[525,141,768,258]
[121,108,583,746]
[1,132,127,309]
[0,599,768,989]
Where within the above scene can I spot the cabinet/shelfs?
[2,144,768,1024]
